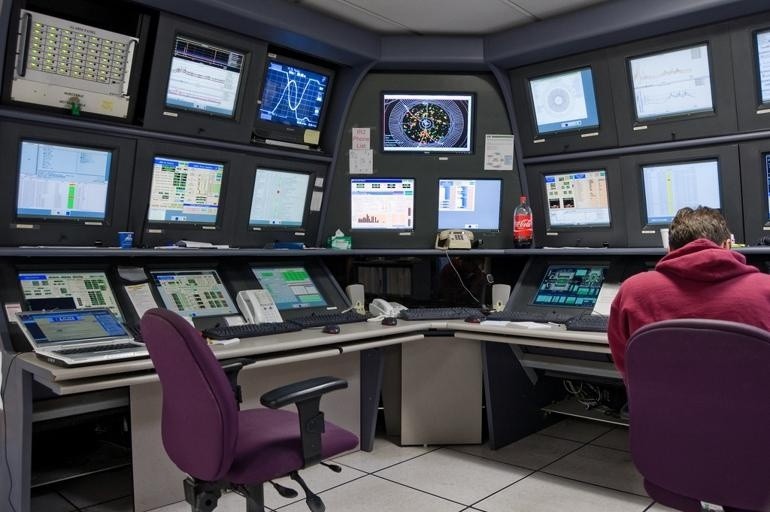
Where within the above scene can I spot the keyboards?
[566,313,609,333]
[203,320,303,340]
[483,311,578,324]
[285,312,368,329]
[400,307,486,322]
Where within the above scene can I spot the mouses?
[464,315,481,323]
[321,324,340,334]
[381,317,398,326]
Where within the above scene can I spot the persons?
[607,205,770,392]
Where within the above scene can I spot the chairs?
[622,317,770,512]
[139,305,360,512]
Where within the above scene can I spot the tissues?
[327,229,352,249]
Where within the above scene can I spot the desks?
[0,316,634,512]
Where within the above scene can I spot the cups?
[118,230,136,249]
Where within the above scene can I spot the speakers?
[492,284,510,314]
[347,284,364,313]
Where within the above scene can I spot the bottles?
[514,197,533,249]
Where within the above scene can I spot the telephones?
[367,299,408,322]
[435,230,474,250]
[236,289,283,324]
[481,274,495,315]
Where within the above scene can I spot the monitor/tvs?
[149,267,240,320]
[756,30,770,102]
[628,40,716,120]
[247,165,312,229]
[9,8,142,121]
[164,32,246,121]
[146,155,225,226]
[348,177,417,235]
[256,60,330,130]
[15,139,113,222]
[379,88,477,157]
[527,66,601,134]
[538,168,613,230]
[532,266,604,308]
[641,158,726,227]
[435,177,504,232]
[14,271,126,325]
[250,265,329,311]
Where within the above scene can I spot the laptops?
[14,306,151,369]
